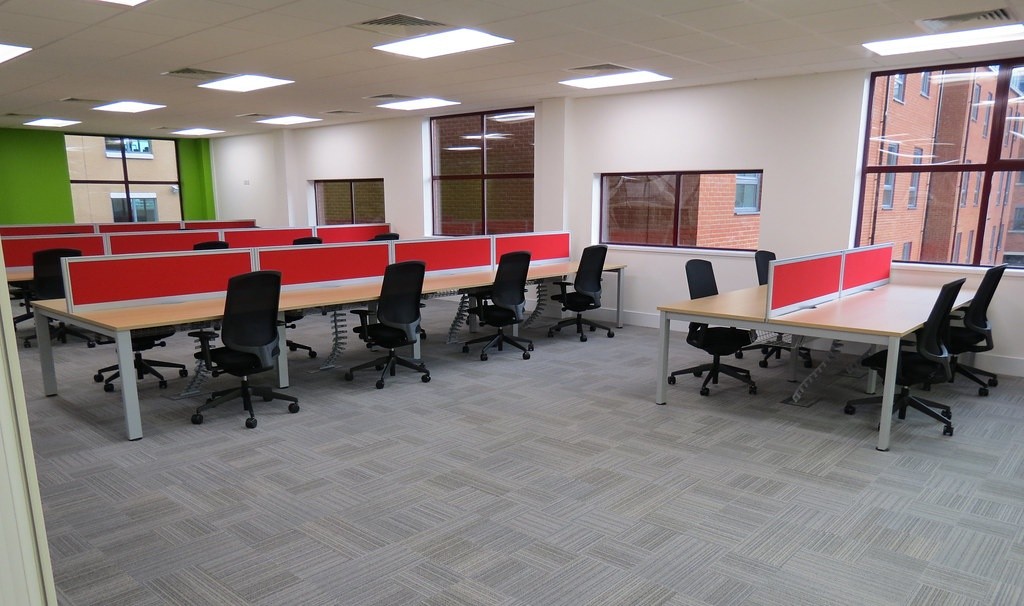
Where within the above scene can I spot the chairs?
[919,262,1007,396]
[11,279,34,330]
[93,326,189,392]
[667,259,757,396]
[843,277,966,436]
[460,251,535,360]
[285,311,319,358]
[344,260,430,389]
[193,241,230,329]
[735,251,814,368]
[24,247,96,349]
[190,269,299,429]
[547,245,614,342]
[294,237,328,315]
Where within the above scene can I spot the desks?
[0,224,391,282]
[653,242,977,451]
[0,219,256,237]
[30,231,580,441]
